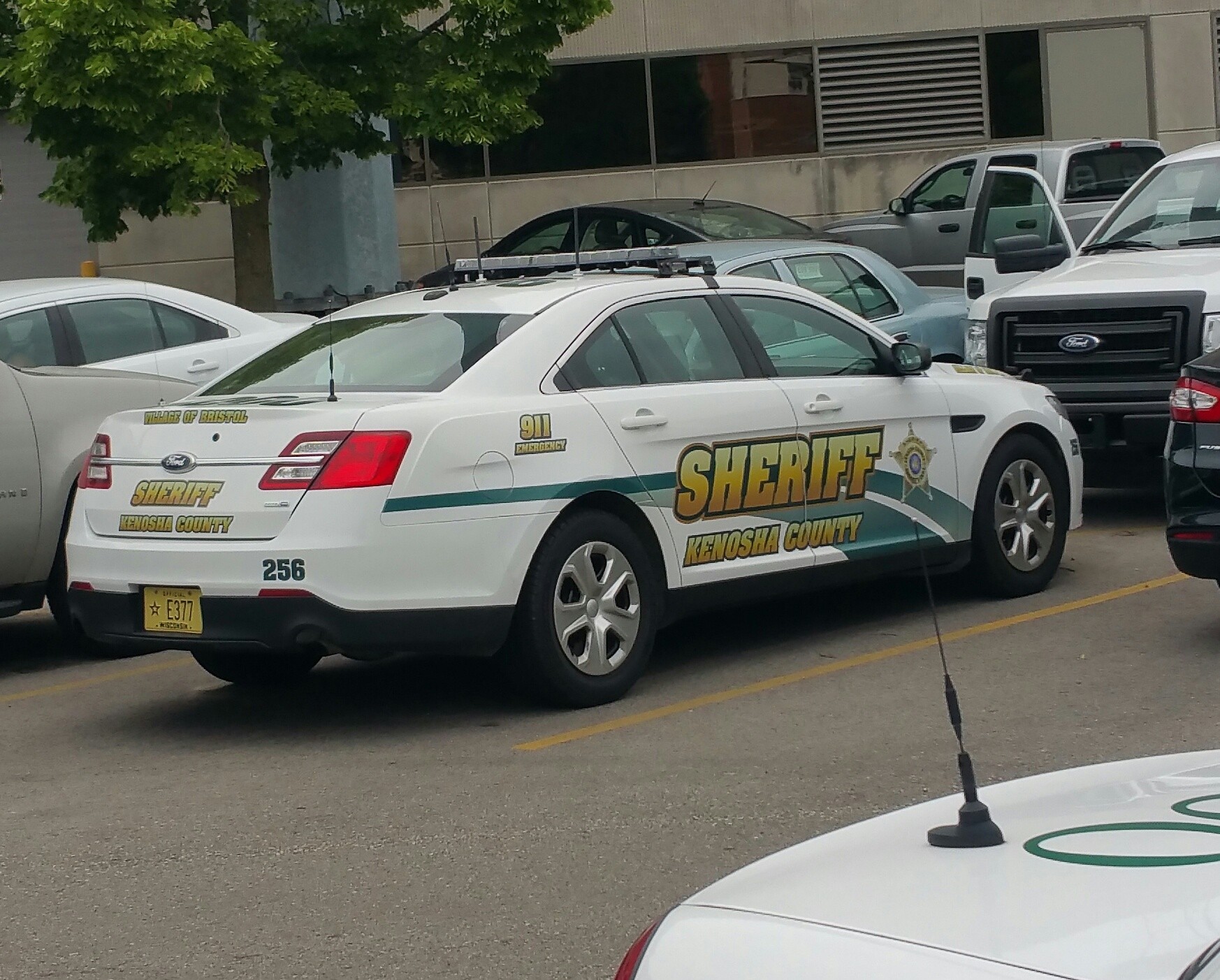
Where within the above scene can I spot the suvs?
[959,135,1220,489]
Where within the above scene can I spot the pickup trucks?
[820,138,1175,292]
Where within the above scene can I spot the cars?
[637,236,978,374]
[0,274,317,661]
[59,266,1091,705]
[1162,349,1220,584]
[609,746,1219,980]
[417,197,845,287]
